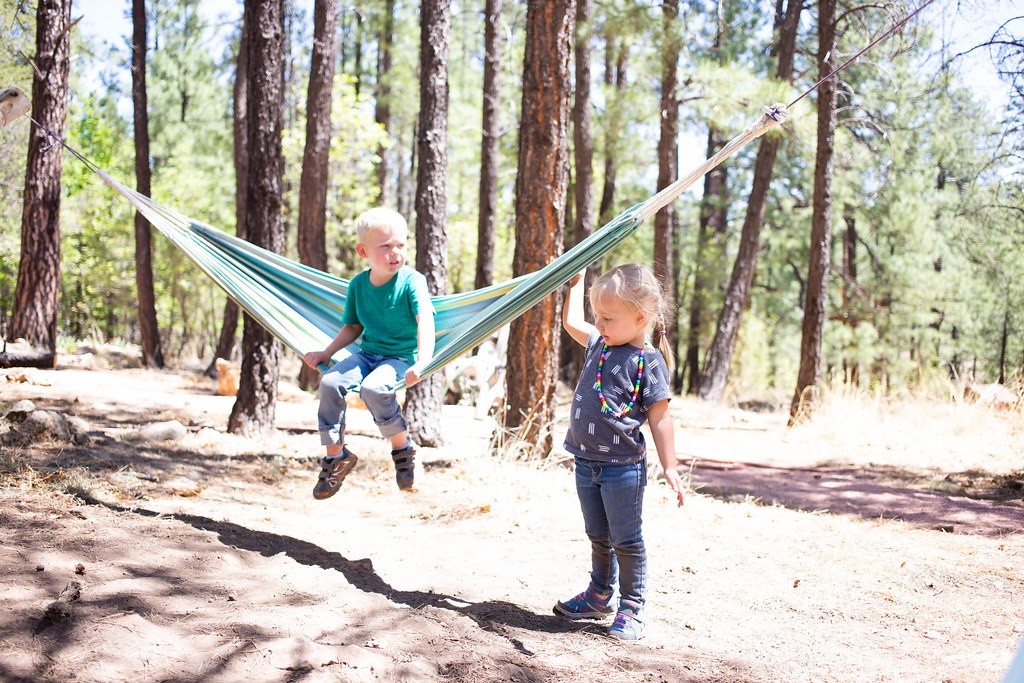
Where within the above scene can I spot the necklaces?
[598,341,647,418]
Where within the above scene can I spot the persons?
[304,205,435,499]
[555,263,684,641]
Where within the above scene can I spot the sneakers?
[392,442,423,491]
[313,444,358,500]
[555,586,615,618]
[607,610,644,641]
